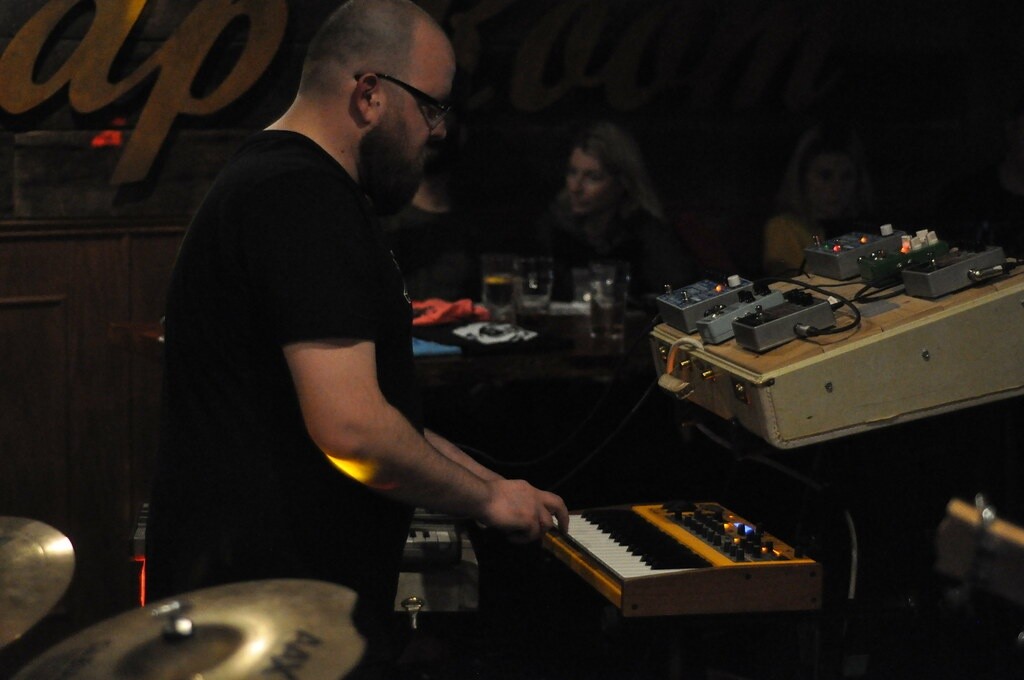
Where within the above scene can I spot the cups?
[480,255,516,336]
[587,259,627,341]
[518,256,556,314]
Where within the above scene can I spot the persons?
[376,92,552,300]
[762,119,877,273]
[145,0,569,680]
[931,98,1023,259]
[553,120,702,286]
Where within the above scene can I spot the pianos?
[521,493,828,623]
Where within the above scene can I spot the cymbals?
[0,512,78,652]
[21,578,373,680]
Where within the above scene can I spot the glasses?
[354,71,454,129]
